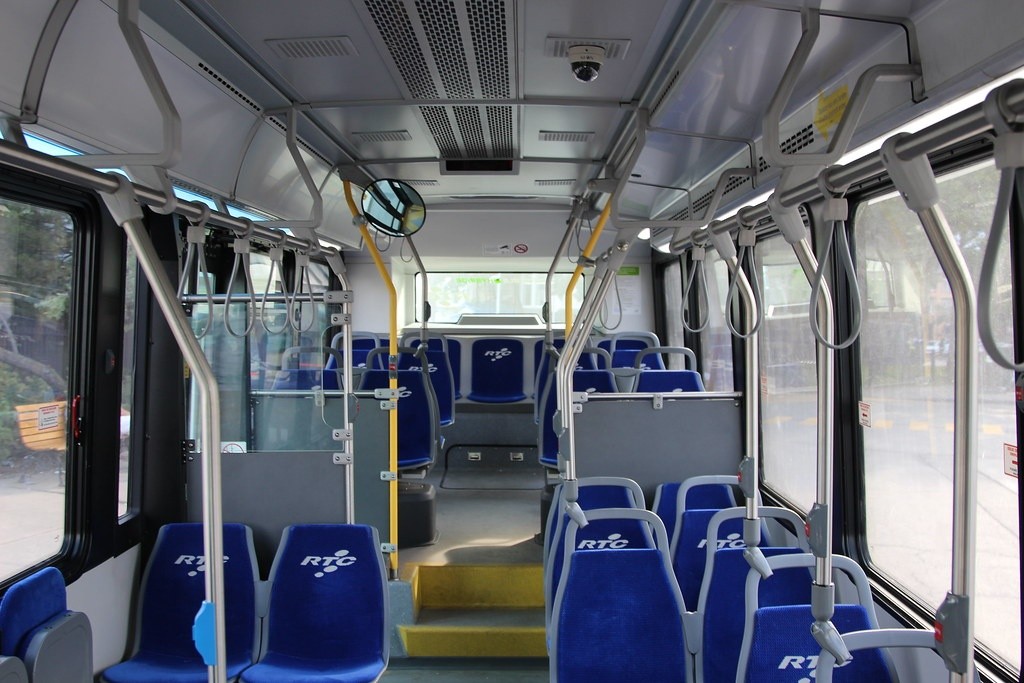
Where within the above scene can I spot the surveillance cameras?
[567,45,605,84]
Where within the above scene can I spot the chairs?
[669,475,774,613]
[102,520,262,683]
[537,347,620,471]
[633,346,706,393]
[549,507,694,683]
[264,346,347,393]
[815,628,984,683]
[734,552,901,683]
[648,481,680,550]
[595,340,649,368]
[695,505,817,683]
[607,331,667,370]
[531,334,584,401]
[398,332,455,429]
[356,346,436,472]
[0,567,97,682]
[544,476,647,632]
[348,333,391,350]
[410,339,461,399]
[239,523,392,683]
[467,338,527,403]
[324,332,386,371]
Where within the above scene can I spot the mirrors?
[360,178,427,238]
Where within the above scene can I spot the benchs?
[12,398,132,488]
